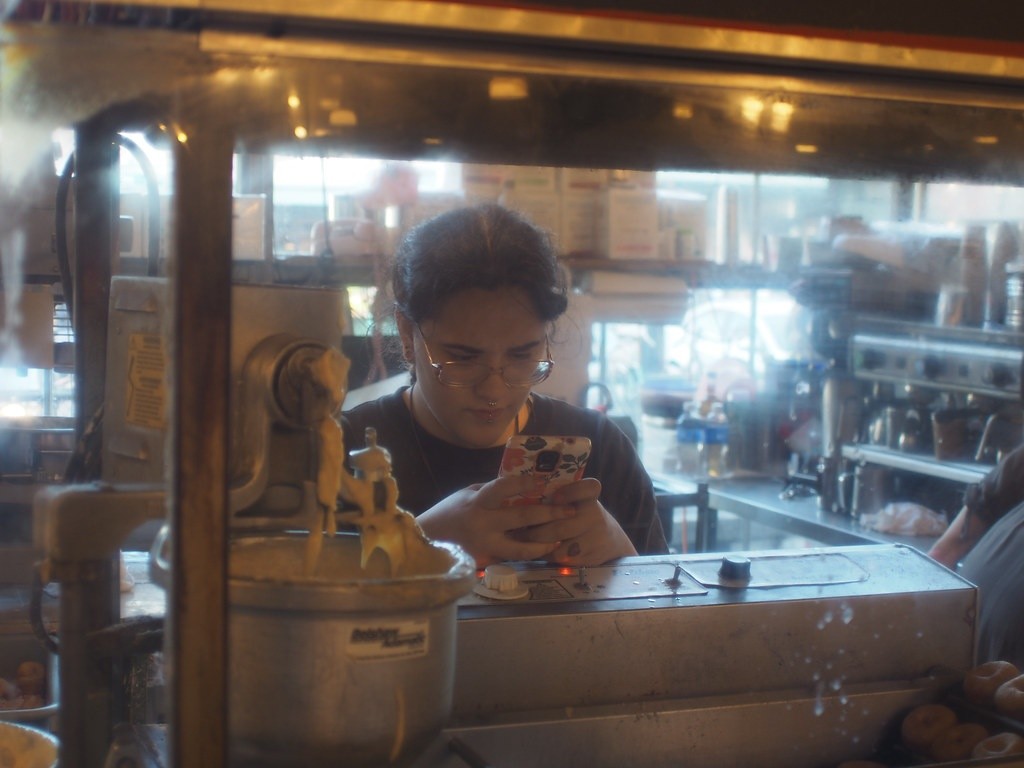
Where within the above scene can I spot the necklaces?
[410,381,519,499]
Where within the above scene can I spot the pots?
[149,525,475,768]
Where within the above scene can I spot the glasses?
[407,311,554,390]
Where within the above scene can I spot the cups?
[838,463,894,518]
[931,408,981,459]
[926,219,1024,331]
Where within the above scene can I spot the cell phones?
[495,435,592,532]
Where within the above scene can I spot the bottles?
[665,371,760,480]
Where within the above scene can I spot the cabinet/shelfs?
[838,319,1024,536]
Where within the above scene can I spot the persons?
[926,443,1024,661]
[354,426,393,482]
[339,202,668,567]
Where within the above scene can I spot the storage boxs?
[120,152,710,260]
